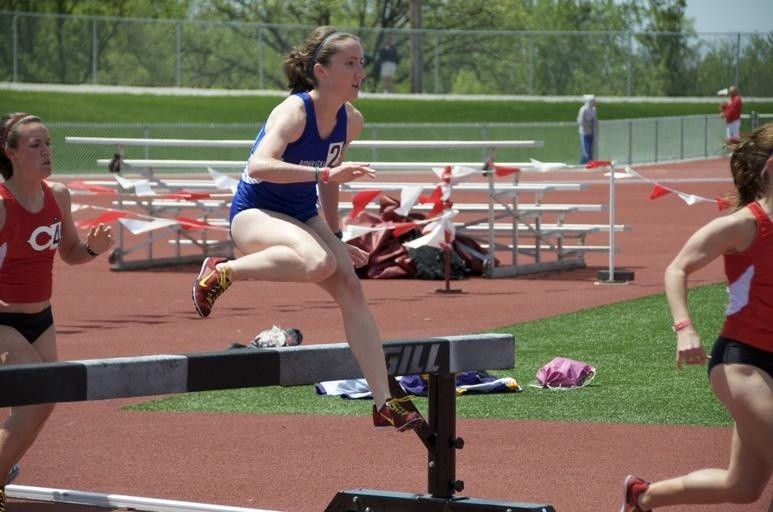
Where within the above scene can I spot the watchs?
[335,229,343,239]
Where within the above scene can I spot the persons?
[577,94,597,164]
[720,86,743,138]
[190,26,423,433]
[620,124,773,512]
[0,112,116,512]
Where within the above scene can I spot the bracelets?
[321,167,329,184]
[315,167,319,183]
[86,245,100,257]
[672,320,692,332]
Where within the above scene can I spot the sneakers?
[373,397,424,432]
[192,257,230,317]
[623,474,652,512]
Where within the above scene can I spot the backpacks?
[527,356,596,390]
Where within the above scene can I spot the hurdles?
[0,333,554,512]
